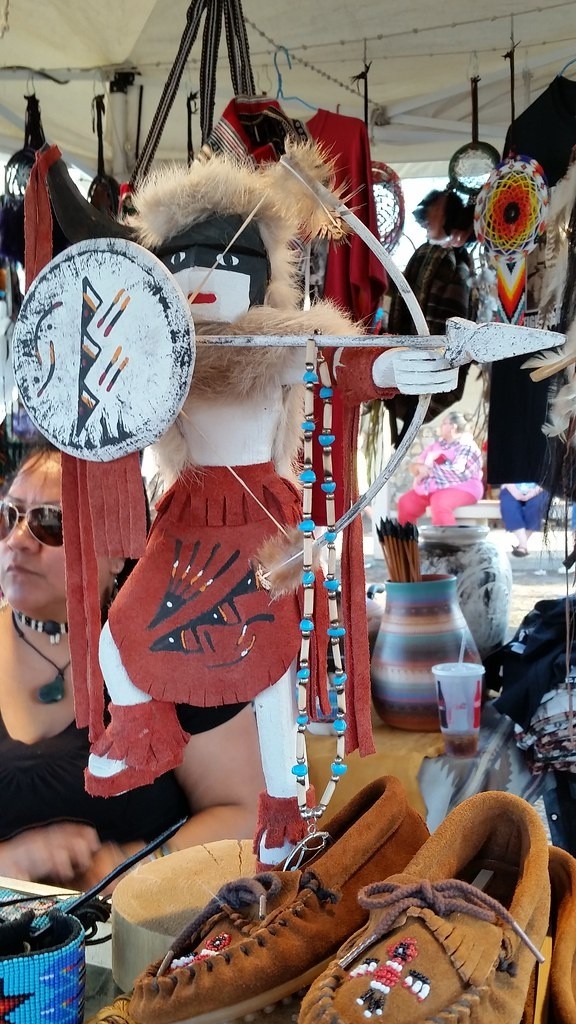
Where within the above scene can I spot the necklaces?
[15,609,67,644]
[12,611,71,703]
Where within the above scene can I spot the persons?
[498,483,551,557]
[572,503,576,545]
[0,439,266,896]
[398,412,484,528]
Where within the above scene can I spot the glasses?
[0,501,64,548]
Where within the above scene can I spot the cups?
[432,663,484,756]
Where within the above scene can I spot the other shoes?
[297,791,551,1024]
[547,844,576,1024]
[129,774,431,1024]
